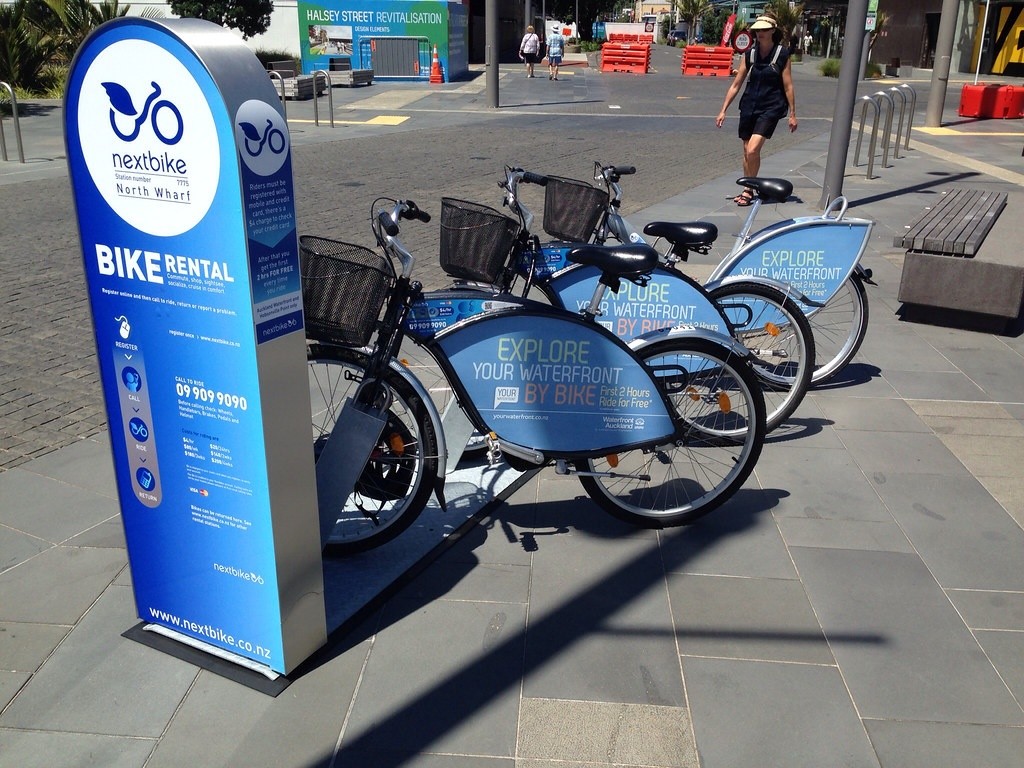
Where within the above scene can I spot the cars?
[666,30,687,46]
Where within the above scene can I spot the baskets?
[299,235,393,347]
[440,197,519,284]
[543,175,609,245]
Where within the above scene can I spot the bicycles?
[298,159,881,560]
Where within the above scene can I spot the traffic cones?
[429,43,444,83]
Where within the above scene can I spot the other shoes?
[525,74,531,78]
[554,77,558,81]
[531,74,535,77]
[549,74,553,80]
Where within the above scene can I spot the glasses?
[755,28,772,33]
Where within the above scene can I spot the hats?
[749,16,777,29]
[551,25,559,32]
[527,26,535,33]
[807,31,809,33]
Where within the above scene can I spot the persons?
[715,14,798,206]
[667,34,674,47]
[546,26,564,80]
[804,31,813,56]
[337,41,344,53]
[519,25,540,78]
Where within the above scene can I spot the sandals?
[738,190,753,205]
[734,194,742,201]
